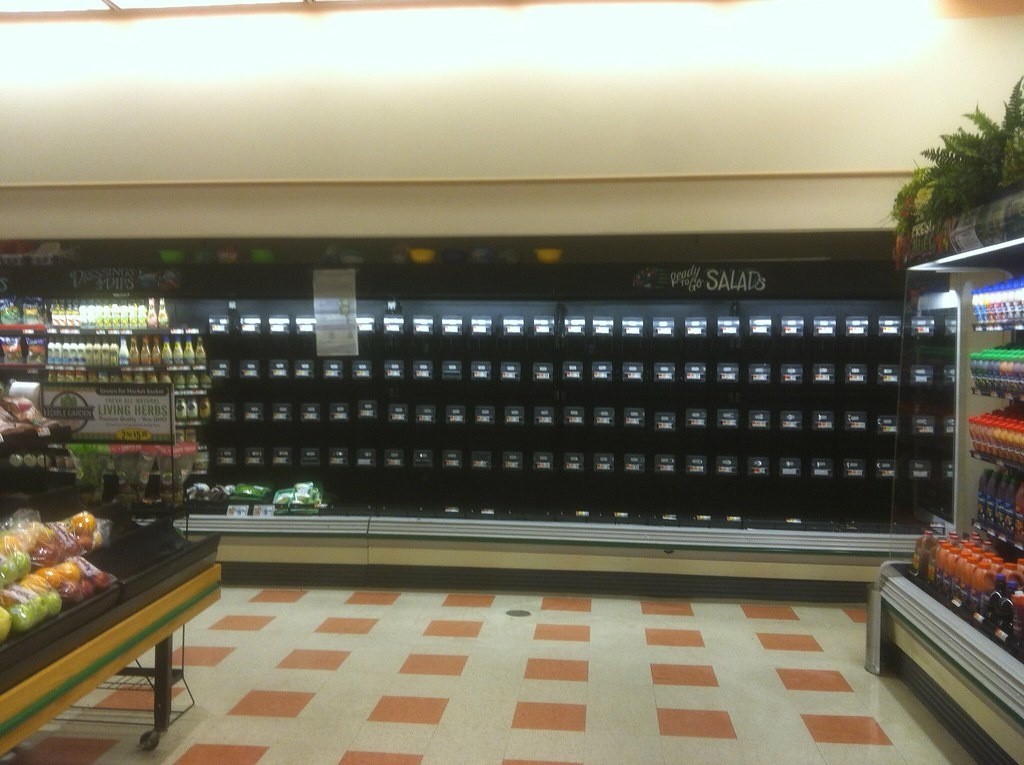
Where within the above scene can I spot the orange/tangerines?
[35,562,83,588]
[70,511,102,550]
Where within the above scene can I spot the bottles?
[46,335,207,366]
[48,367,212,389]
[969,342,1024,401]
[193,446,210,474]
[46,443,80,473]
[49,297,169,328]
[971,277,1024,331]
[978,468,1024,546]
[912,529,1024,642]
[173,390,215,420]
[969,406,1024,463]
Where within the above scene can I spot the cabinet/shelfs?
[0,325,209,522]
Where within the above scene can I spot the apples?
[57,571,110,604]
[27,522,92,568]
[7,589,62,633]
[0,550,31,589]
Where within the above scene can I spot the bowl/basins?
[159,249,185,264]
[534,249,562,262]
[410,249,435,261]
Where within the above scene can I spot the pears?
[0,606,13,643]
